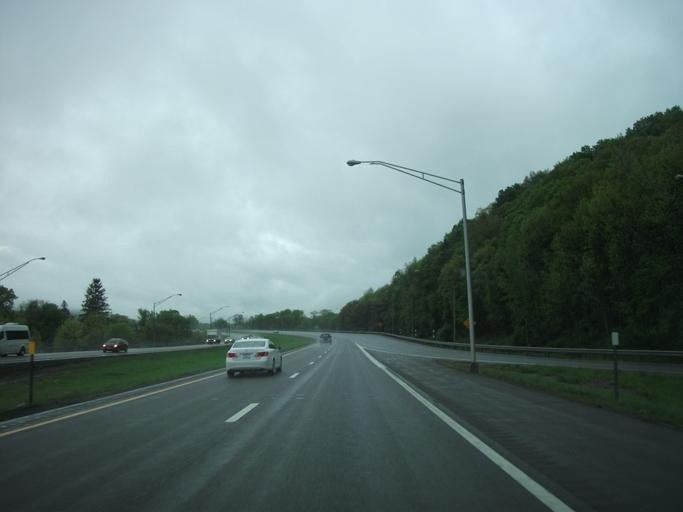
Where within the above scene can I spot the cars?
[317,332,333,345]
[99,338,128,352]
[223,329,282,377]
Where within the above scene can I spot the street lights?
[208,305,244,336]
[151,293,181,347]
[0,257,45,281]
[345,160,475,362]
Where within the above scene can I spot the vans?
[1,322,32,356]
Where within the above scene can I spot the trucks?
[206,329,220,344]
